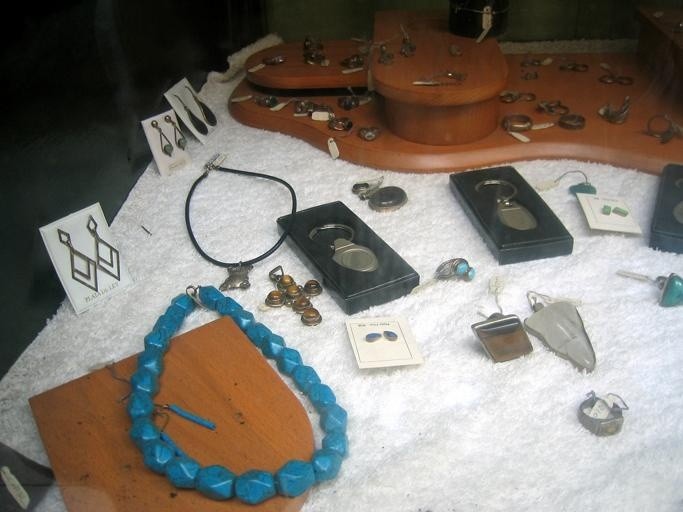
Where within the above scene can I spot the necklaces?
[127,286,350,504]
[170,153,297,291]
